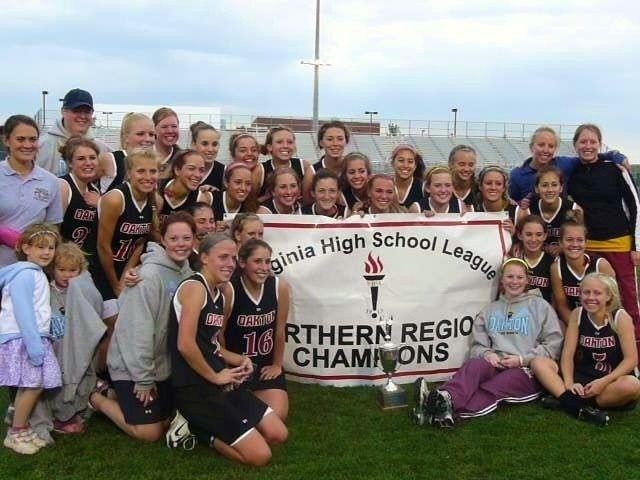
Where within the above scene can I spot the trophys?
[367,333,412,409]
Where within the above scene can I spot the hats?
[60,89,94,113]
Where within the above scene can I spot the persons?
[0,222,64,457]
[167,232,291,467]
[411,258,565,432]
[529,271,638,424]
[0,89,638,435]
[215,241,288,425]
[76,214,197,442]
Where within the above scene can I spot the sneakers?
[412,376,432,425]
[52,418,85,438]
[166,409,197,451]
[80,392,96,424]
[95,379,108,394]
[427,389,454,430]
[34,424,54,444]
[26,427,48,447]
[579,405,611,427]
[4,429,39,455]
[541,397,559,408]
[4,407,15,425]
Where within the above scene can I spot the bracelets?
[516,354,525,369]
